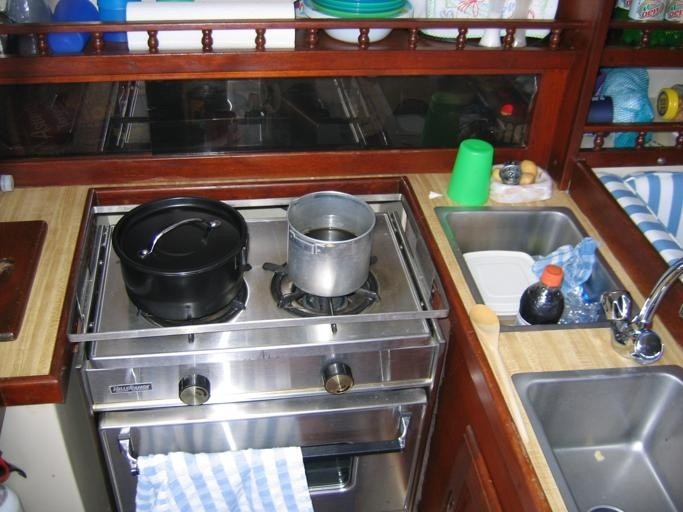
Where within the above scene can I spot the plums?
[499,165,521,184]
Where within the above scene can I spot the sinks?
[510,366,683,512]
[433,205,643,332]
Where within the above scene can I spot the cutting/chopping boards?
[0,219,49,344]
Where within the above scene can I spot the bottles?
[3,0,50,57]
[609,0,682,47]
[514,264,565,326]
[487,103,516,145]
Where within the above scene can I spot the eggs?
[520,174,534,185]
[520,159,537,177]
[492,168,503,183]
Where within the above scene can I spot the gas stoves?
[66,203,454,410]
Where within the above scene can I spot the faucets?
[600,255,683,362]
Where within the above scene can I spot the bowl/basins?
[299,0,415,43]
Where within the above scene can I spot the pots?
[107,194,251,323]
[284,190,377,299]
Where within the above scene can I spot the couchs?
[596,169,682,283]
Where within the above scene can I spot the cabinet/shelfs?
[0,17,594,182]
[415,339,553,512]
[569,18,682,167]
[469,118,472,126]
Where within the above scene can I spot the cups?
[43,0,98,54]
[96,0,143,44]
[444,136,494,208]
[585,98,613,137]
[419,91,468,147]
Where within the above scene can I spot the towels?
[135,444,317,512]
